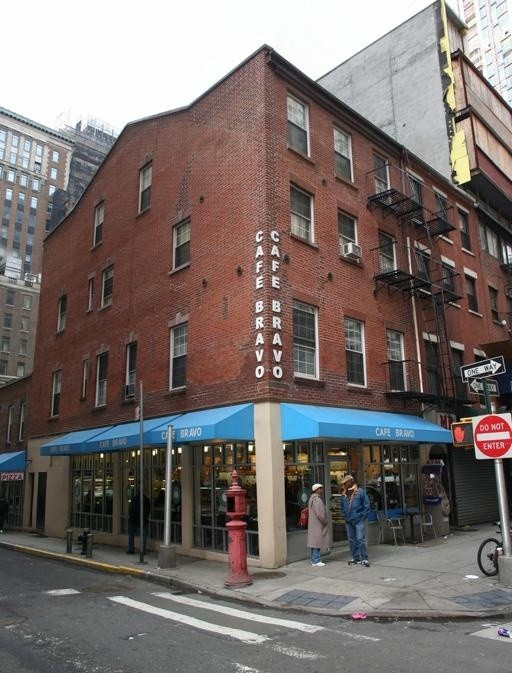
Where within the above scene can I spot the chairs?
[376,507,437,546]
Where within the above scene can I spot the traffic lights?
[450,417,472,448]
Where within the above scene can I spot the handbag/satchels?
[300,508,309,526]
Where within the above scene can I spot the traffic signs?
[460,355,506,397]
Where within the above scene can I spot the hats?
[341,475,354,484]
[312,484,323,491]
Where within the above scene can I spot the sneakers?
[348,559,361,565]
[362,560,369,566]
[310,560,326,567]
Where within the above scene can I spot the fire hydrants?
[78,527,90,555]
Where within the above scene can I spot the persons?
[125,488,150,555]
[0,495,9,534]
[306,483,333,567]
[340,475,371,568]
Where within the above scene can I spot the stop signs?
[472,413,512,460]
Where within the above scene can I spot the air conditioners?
[344,243,363,259]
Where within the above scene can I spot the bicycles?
[478,521,512,576]
